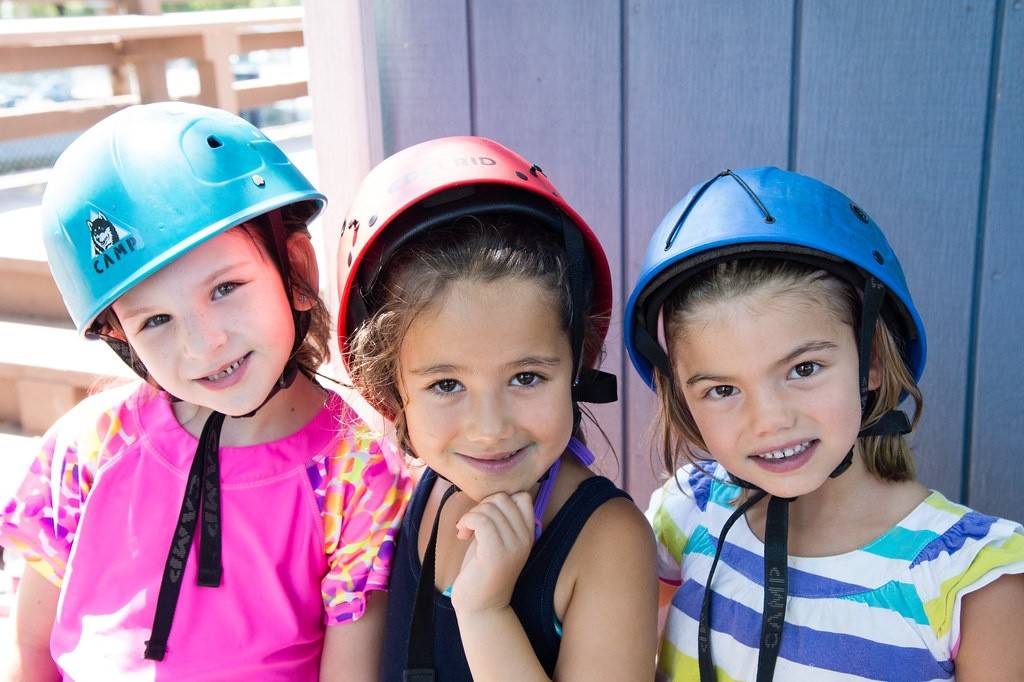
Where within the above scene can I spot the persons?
[0,102,416,682]
[623,165,1024,682]
[336,135,659,682]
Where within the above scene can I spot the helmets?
[38,100,329,341]
[333,135,614,421]
[622,165,928,435]
